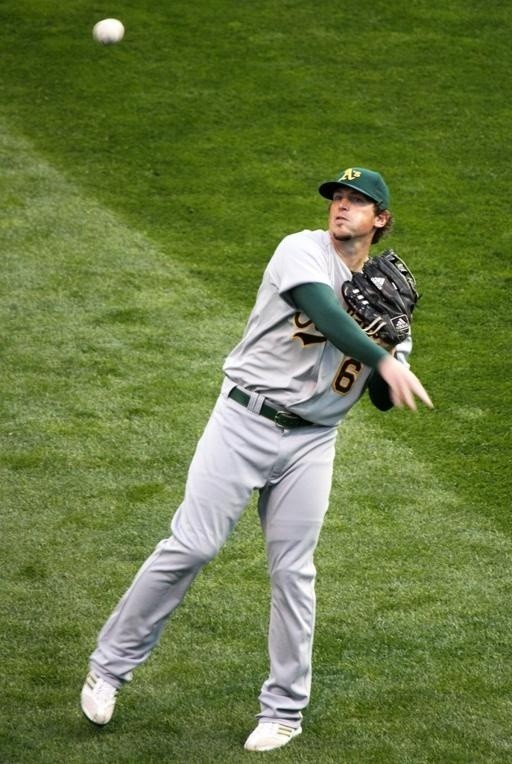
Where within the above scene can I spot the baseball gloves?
[343,248,415,343]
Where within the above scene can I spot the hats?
[317,167,390,211]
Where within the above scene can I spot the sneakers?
[243,719,305,752]
[79,669,120,726]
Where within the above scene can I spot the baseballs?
[94,18,125,46]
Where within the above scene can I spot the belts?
[228,387,315,429]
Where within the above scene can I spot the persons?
[79,164,436,753]
